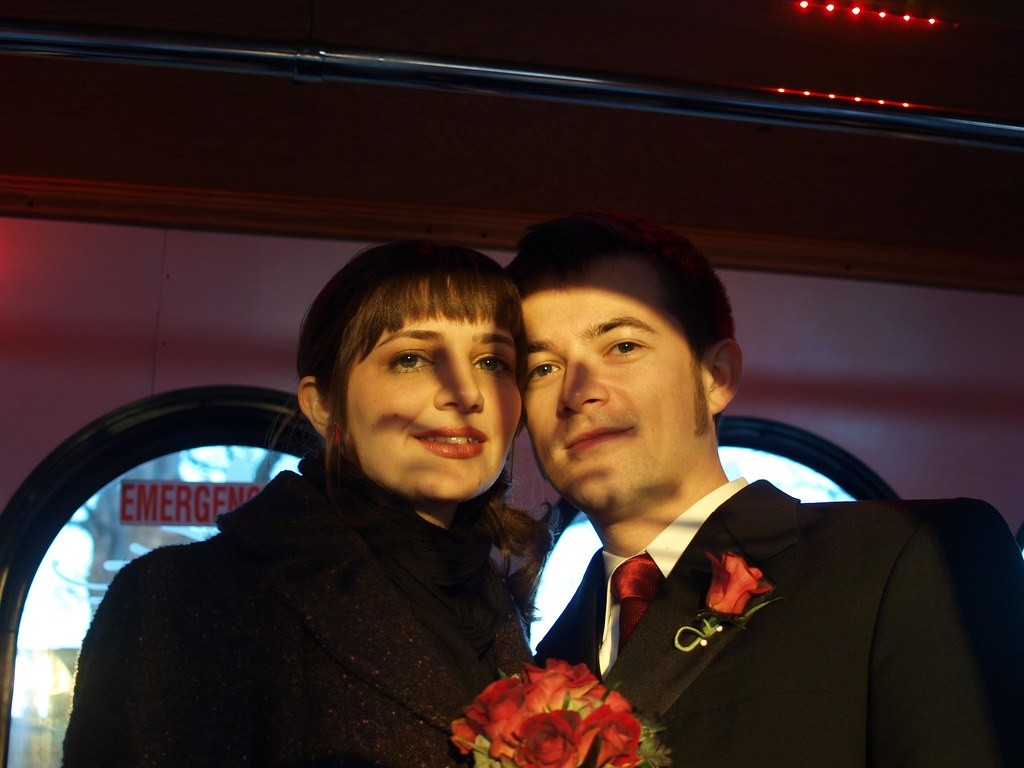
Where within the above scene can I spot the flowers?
[450,658,674,768]
[674,544,785,651]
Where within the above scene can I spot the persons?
[63,239,556,768]
[505,210,1024,767]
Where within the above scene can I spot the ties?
[610,552,664,662]
